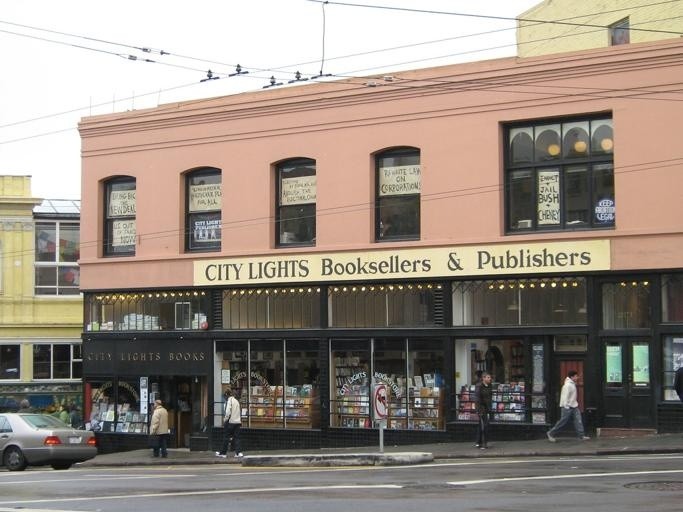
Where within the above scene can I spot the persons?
[16,399,80,428]
[149,400,169,458]
[216,389,243,458]
[474,372,493,450]
[674,367,683,401]
[546,370,592,441]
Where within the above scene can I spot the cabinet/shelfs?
[331,347,445,430]
[237,384,320,428]
[458,341,525,421]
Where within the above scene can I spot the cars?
[0,412,98,471]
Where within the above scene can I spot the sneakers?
[577,436,591,441]
[215,452,228,458]
[546,431,557,442]
[234,453,244,458]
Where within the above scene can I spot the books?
[458,383,525,422]
[87,313,162,331]
[337,374,440,429]
[192,313,208,329]
[86,402,148,433]
[241,384,312,419]
